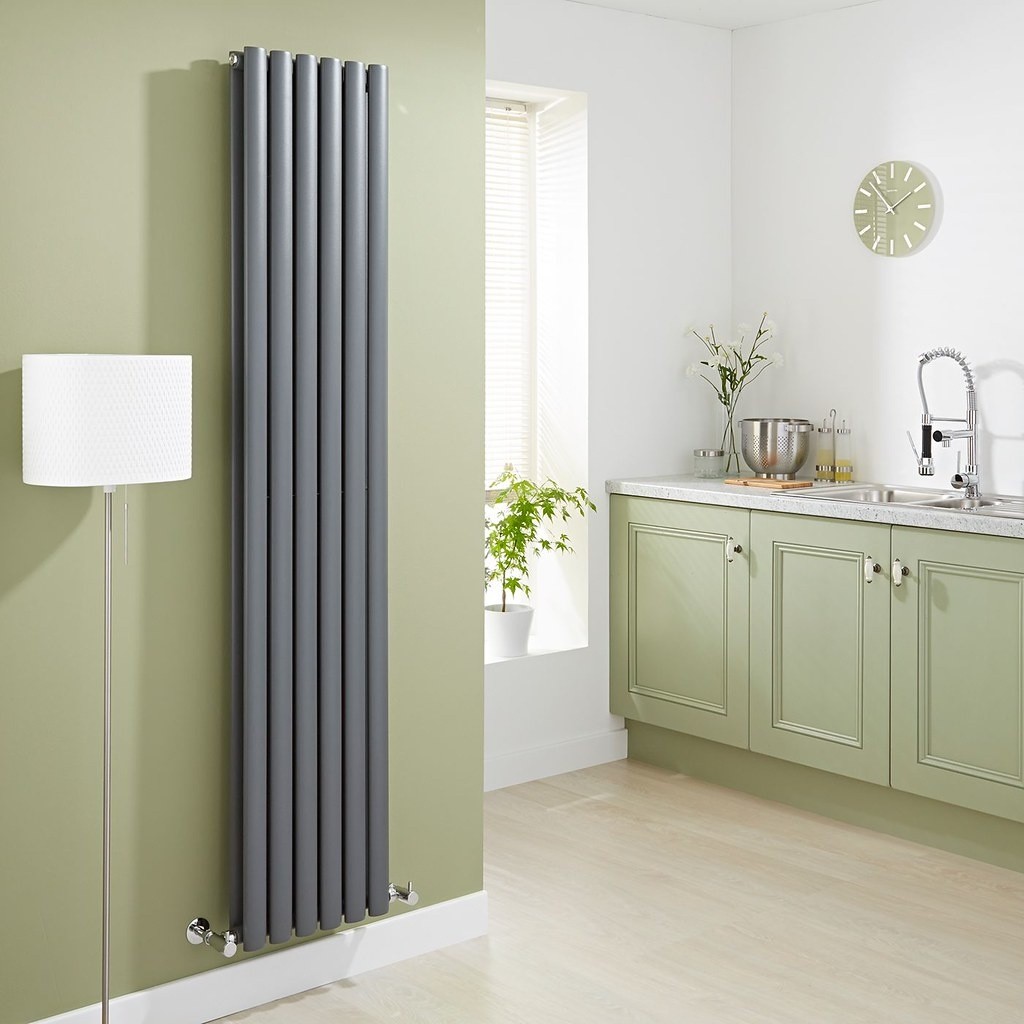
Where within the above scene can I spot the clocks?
[853,161,936,257]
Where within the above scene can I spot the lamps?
[20,351,192,1024]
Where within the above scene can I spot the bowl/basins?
[737,418,813,480]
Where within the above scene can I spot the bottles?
[835,419,852,481]
[816,418,834,480]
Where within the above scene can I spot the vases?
[713,392,745,476]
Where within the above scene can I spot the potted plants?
[484,463,597,657]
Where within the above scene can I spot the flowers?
[681,310,784,473]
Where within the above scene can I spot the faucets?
[905,346,978,477]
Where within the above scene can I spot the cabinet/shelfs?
[749,507,1024,875]
[609,493,750,792]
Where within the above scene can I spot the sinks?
[771,482,964,508]
